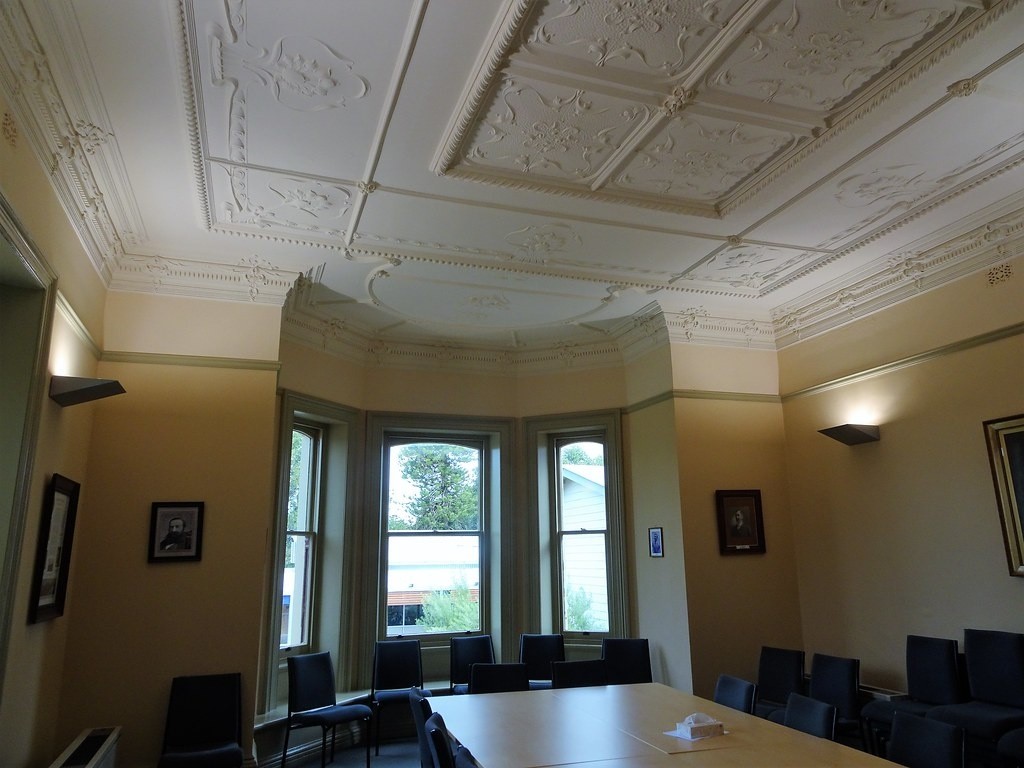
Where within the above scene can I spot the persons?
[160,517,192,550]
[652,533,661,553]
[730,508,752,537]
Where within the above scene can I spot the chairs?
[925,627,1024,768]
[407,685,458,768]
[281,651,373,768]
[766,653,864,742]
[450,635,498,696]
[783,691,838,742]
[598,637,655,686]
[712,673,758,716]
[517,632,568,691]
[156,671,246,768]
[366,638,433,758]
[883,710,968,767]
[859,632,967,759]
[550,659,609,690]
[751,645,808,719]
[425,711,478,768]
[467,662,531,694]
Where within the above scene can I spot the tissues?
[674,712,725,741]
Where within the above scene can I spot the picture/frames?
[982,413,1024,578]
[26,472,83,627]
[715,489,767,556]
[648,527,665,558]
[147,501,205,566]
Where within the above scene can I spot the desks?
[421,681,912,768]
